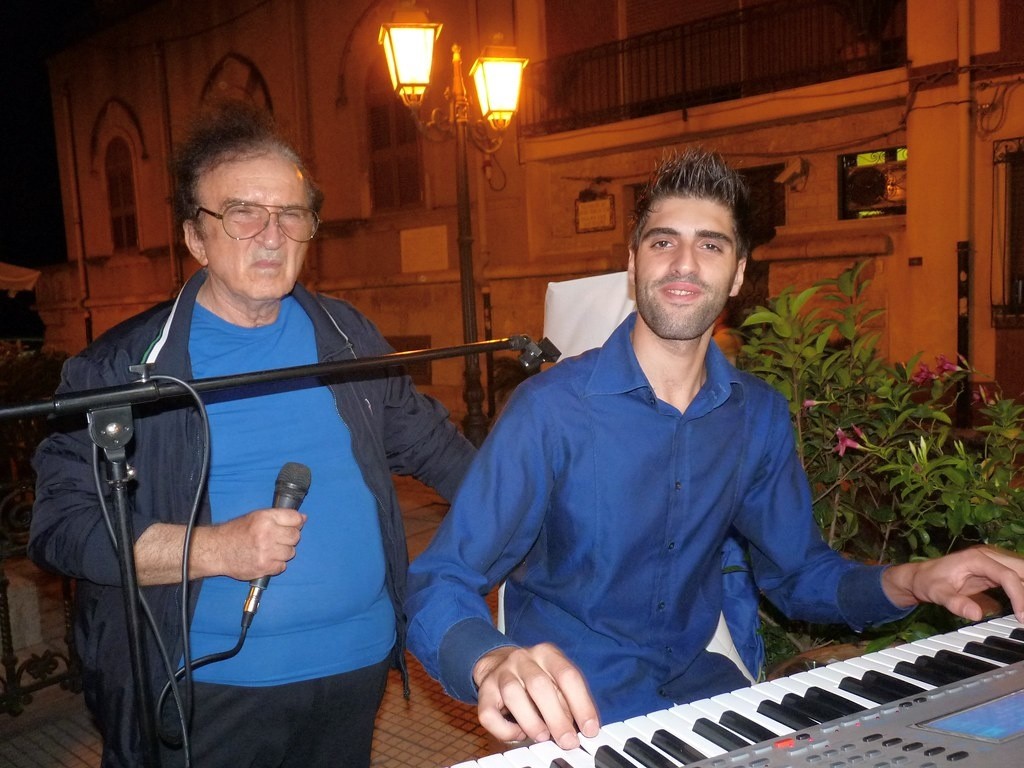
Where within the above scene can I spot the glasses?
[194,201,322,244]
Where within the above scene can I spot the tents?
[1,263,40,297]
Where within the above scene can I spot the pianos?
[452,609,1023,768]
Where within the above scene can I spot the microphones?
[241,462,311,627]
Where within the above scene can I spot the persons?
[26,101,481,768]
[404,149,1024,749]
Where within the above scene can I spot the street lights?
[377,7,529,450]
